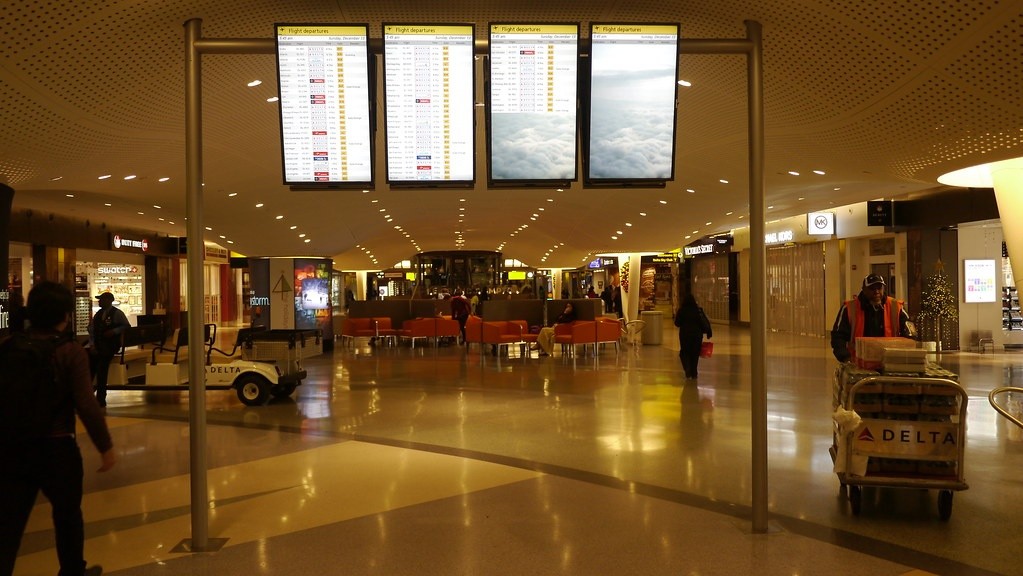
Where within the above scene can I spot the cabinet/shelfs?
[1002,287,1023,331]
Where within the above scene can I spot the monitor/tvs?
[274,21,682,190]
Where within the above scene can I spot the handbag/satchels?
[698,338,713,358]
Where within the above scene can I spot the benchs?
[205,326,266,367]
[112,323,168,365]
[149,323,216,365]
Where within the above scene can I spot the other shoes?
[58,560,103,576]
[530,346,540,350]
[539,353,549,356]
[98,400,107,407]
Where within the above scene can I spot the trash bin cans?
[640,310,664,345]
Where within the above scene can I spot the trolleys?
[828,359,972,521]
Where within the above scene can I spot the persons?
[0,279,115,576]
[531,303,576,357]
[830,273,917,363]
[451,289,471,345]
[587,286,595,298]
[251,305,264,326]
[441,288,451,300]
[673,293,711,380]
[600,286,623,318]
[86,291,131,408]
[470,287,488,315]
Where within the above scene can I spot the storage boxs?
[833,334,961,422]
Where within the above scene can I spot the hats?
[95,292,114,301]
[863,272,886,288]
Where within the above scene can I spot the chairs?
[340,316,623,371]
